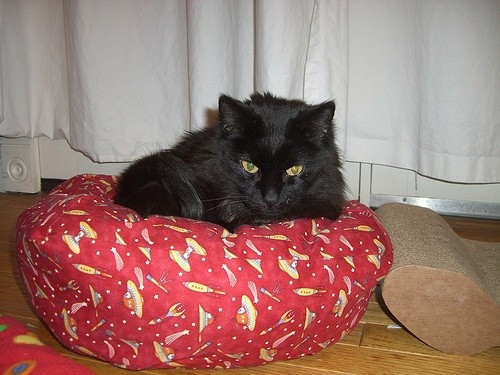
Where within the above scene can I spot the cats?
[111,90,349,231]
